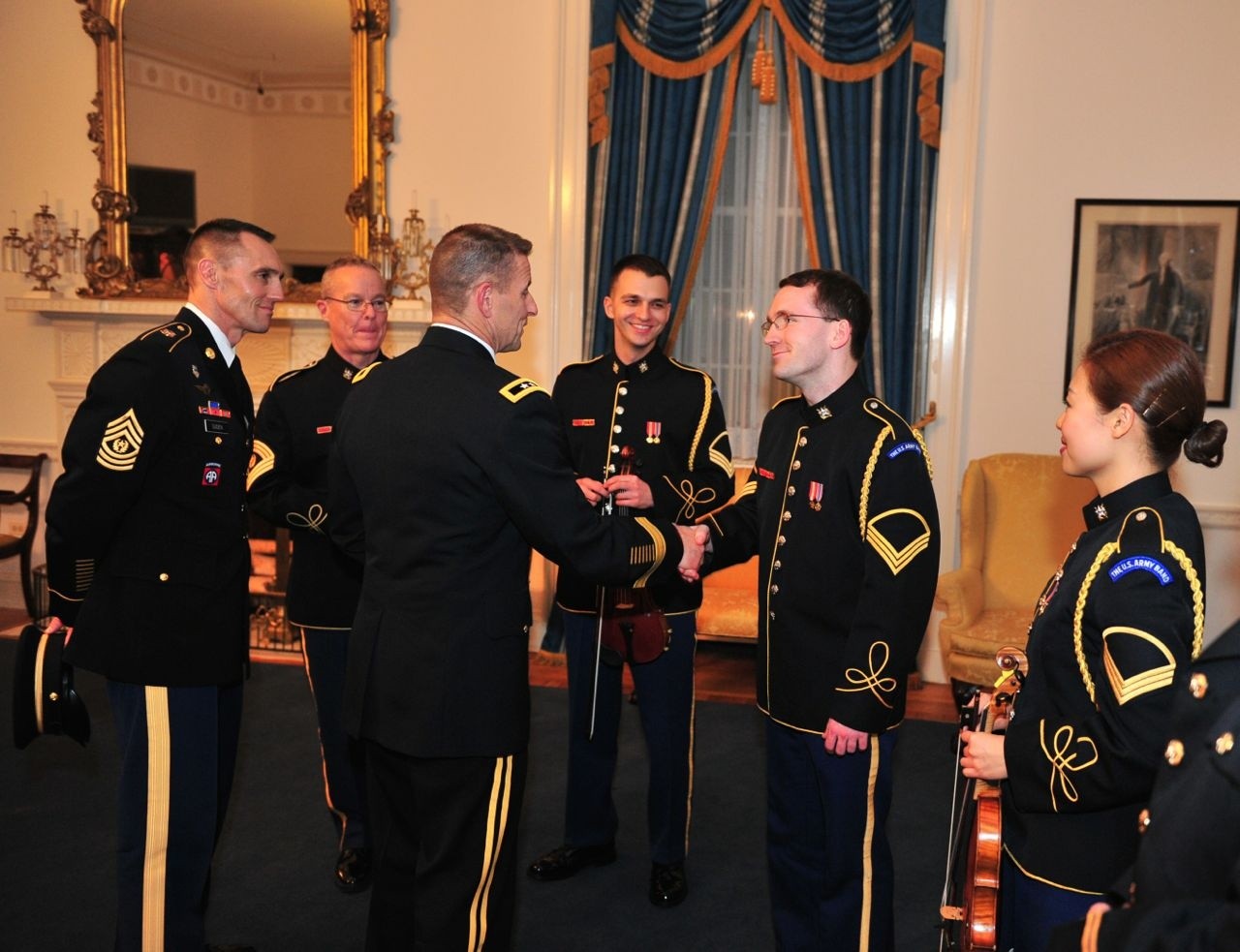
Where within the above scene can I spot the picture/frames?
[1061,197,1240,409]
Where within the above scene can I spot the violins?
[939,646,1027,951]
[594,442,674,667]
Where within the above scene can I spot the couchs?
[532,463,765,648]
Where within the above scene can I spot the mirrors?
[77,0,394,306]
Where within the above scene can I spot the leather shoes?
[335,846,371,894]
[650,864,688,907]
[527,838,617,879]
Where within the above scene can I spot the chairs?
[0,450,50,626]
[933,453,1099,728]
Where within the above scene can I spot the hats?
[11,623,85,749]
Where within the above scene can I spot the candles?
[376,213,384,233]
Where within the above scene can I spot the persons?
[45,218,285,952]
[328,223,703,952]
[528,254,734,907]
[245,259,392,890]
[959,326,1240,952]
[683,268,940,952]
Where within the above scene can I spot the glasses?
[324,295,392,312]
[761,310,840,337]
